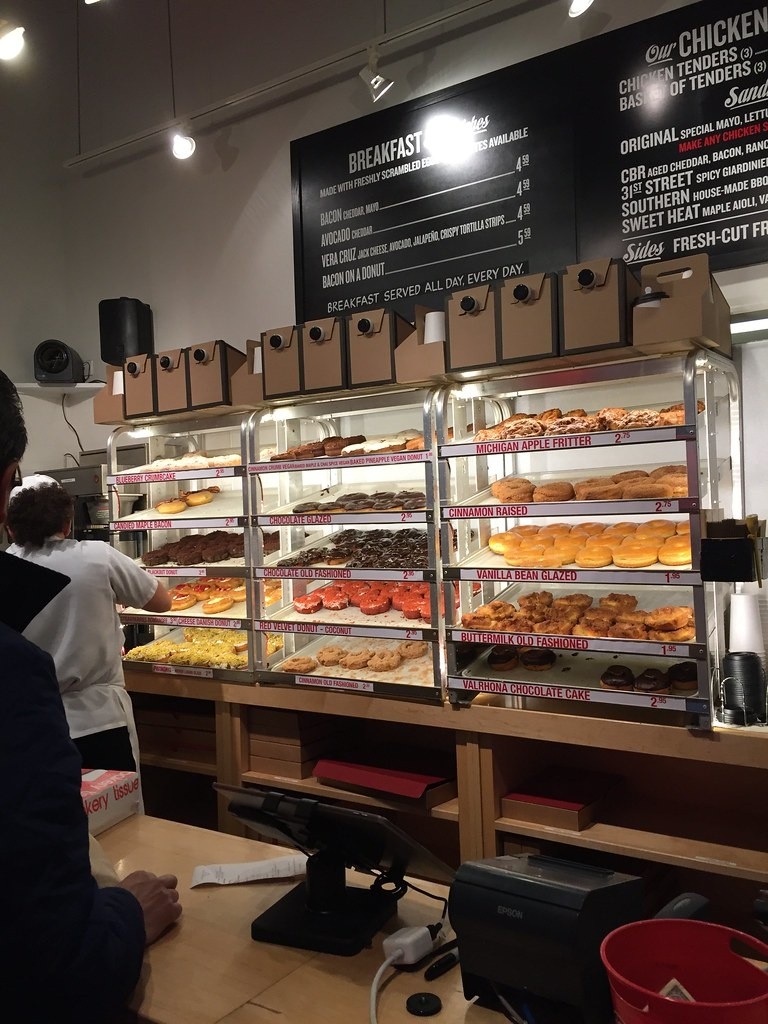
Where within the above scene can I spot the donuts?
[487,644,697,696]
[491,464,689,503]
[267,422,473,674]
[124,446,310,674]
[488,521,691,568]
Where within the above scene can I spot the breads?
[473,400,705,441]
[461,591,695,642]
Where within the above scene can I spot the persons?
[0,373,182,1024]
[3,474,173,815]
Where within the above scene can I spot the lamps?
[170,133,197,159]
[566,0,595,18]
[359,43,395,102]
[0,20,25,59]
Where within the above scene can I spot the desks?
[92,813,507,1023]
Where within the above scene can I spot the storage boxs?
[80,768,143,838]
[92,253,734,426]
[144,709,620,856]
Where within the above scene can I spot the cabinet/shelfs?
[434,350,745,734]
[248,387,505,701]
[125,670,767,935]
[106,408,335,681]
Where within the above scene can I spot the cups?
[729,588,768,693]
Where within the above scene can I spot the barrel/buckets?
[600,919,768,1024]
